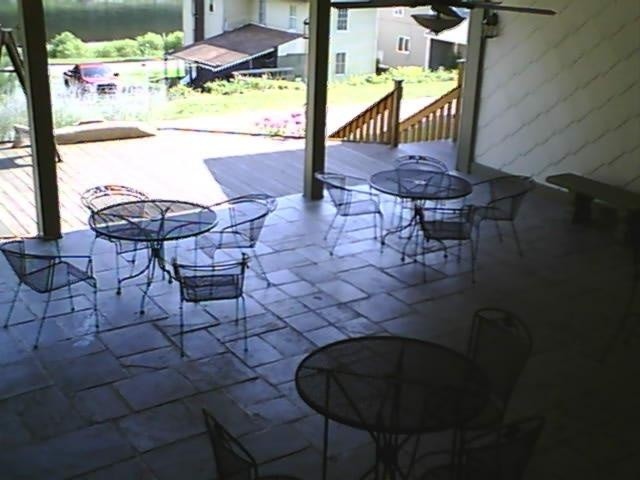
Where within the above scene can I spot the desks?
[295,335,491,480]
[88,199,220,317]
[369,170,473,262]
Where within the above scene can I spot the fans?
[324,0,556,17]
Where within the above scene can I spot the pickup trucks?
[58,61,131,101]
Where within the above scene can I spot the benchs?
[544,172,640,243]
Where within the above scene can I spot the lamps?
[409,13,466,35]
[481,12,500,39]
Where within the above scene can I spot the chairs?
[171,251,252,358]
[469,175,537,257]
[0,240,99,350]
[312,170,386,257]
[80,184,165,296]
[193,191,279,295]
[413,204,480,285]
[406,306,534,477]
[201,407,300,479]
[417,414,546,479]
[392,154,448,229]
